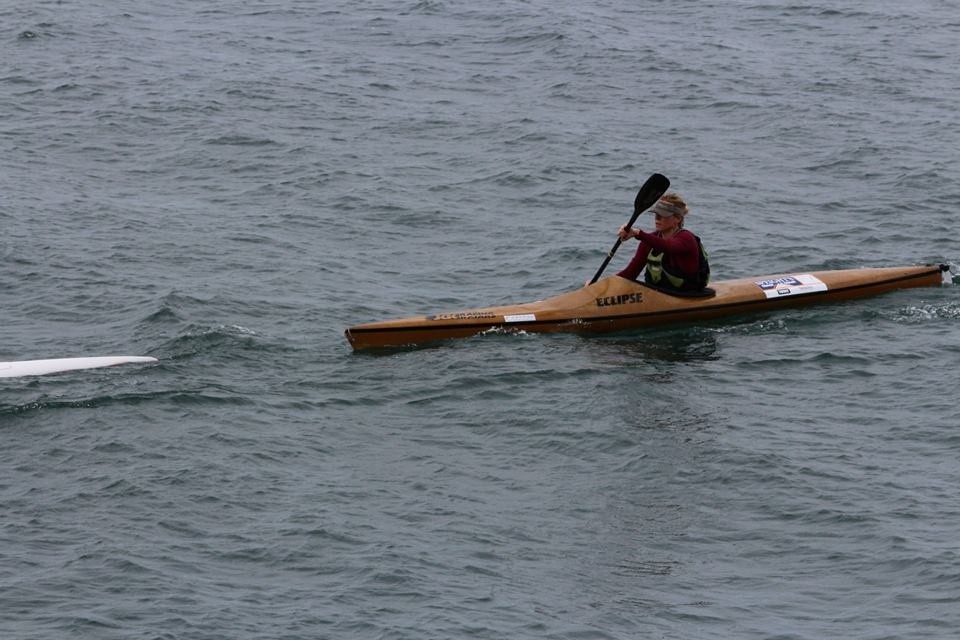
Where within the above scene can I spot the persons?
[584,193,711,296]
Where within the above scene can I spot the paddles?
[589,172,671,285]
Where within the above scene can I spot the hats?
[648,200,688,217]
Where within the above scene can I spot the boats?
[0,353,157,378]
[342,259,951,343]
